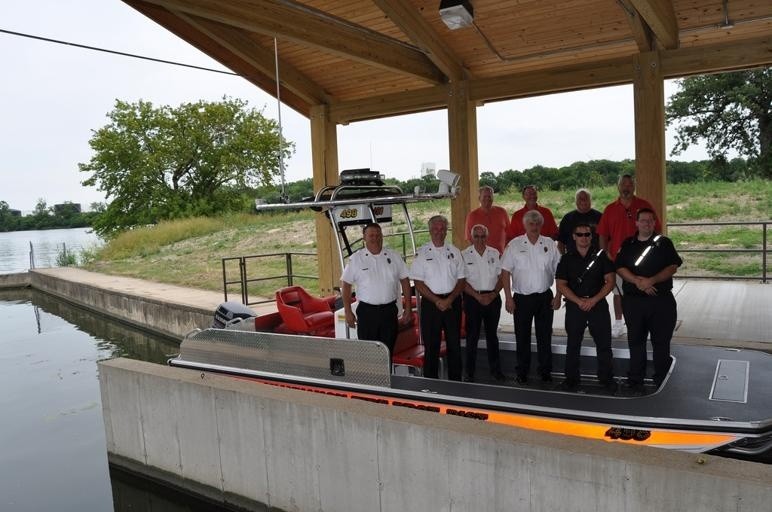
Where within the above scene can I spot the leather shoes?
[491,367,644,391]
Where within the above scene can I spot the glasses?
[575,233,591,237]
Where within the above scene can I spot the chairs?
[391,311,447,378]
[275,285,335,333]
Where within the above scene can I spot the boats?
[168,166,772,455]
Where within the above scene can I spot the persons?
[554,219,616,392]
[615,207,683,385]
[339,223,412,373]
[556,188,604,257]
[594,172,662,340]
[409,215,466,382]
[460,223,503,383]
[499,209,563,385]
[462,185,511,261]
[502,184,560,250]
